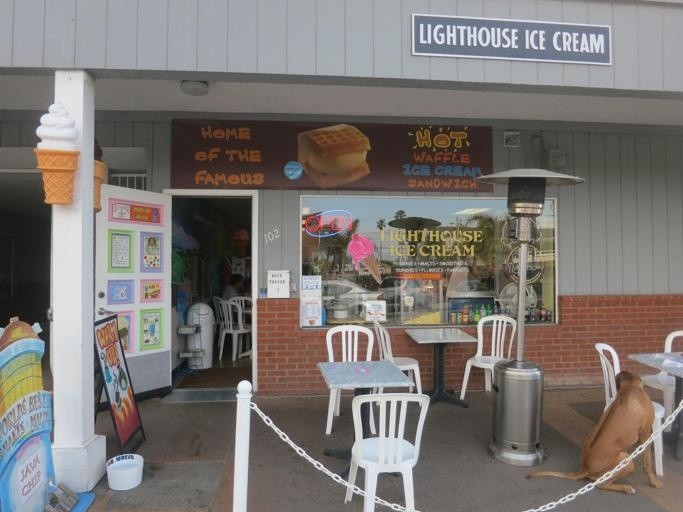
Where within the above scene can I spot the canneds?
[450,302,470,325]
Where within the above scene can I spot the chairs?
[213,284,252,362]
[323,325,376,436]
[344,393,430,512]
[593,342,665,476]
[641,331,680,433]
[460,315,517,402]
[372,321,423,408]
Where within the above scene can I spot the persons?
[146,237,157,248]
[242,279,251,308]
[220,273,244,322]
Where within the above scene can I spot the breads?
[297,124,372,189]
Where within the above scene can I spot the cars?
[371,273,542,303]
[317,278,386,319]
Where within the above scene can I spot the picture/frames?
[231,258,246,278]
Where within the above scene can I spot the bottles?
[449,302,552,325]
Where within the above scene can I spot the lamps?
[180,80,209,96]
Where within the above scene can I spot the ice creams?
[33,99,80,205]
[347,233,382,285]
[94,137,106,212]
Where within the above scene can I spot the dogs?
[525,370,664,495]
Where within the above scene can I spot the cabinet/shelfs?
[173,292,205,376]
